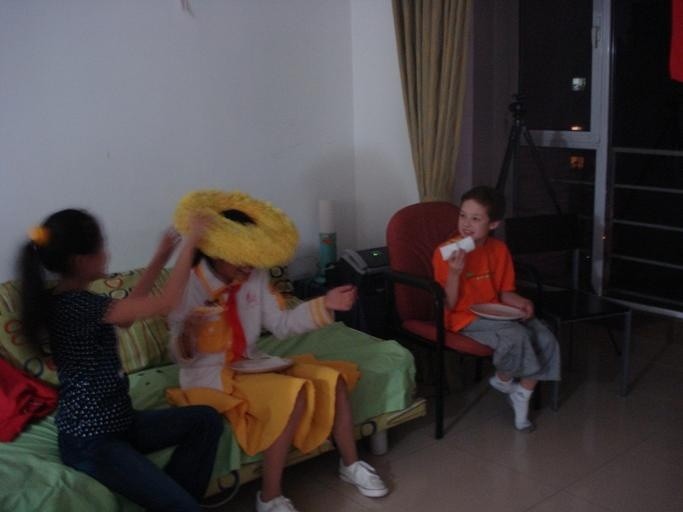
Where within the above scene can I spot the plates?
[229,355,294,373]
[468,303,526,322]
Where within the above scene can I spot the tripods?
[497,118,624,356]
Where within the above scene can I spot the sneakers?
[336,455,390,499]
[254,488,300,512]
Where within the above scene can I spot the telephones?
[341,247,372,278]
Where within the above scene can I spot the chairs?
[500,206,637,411]
[370,196,553,440]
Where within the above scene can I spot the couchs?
[0,262,435,512]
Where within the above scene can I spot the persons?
[433,185,566,432]
[172,208,391,511]
[16,209,228,511]
